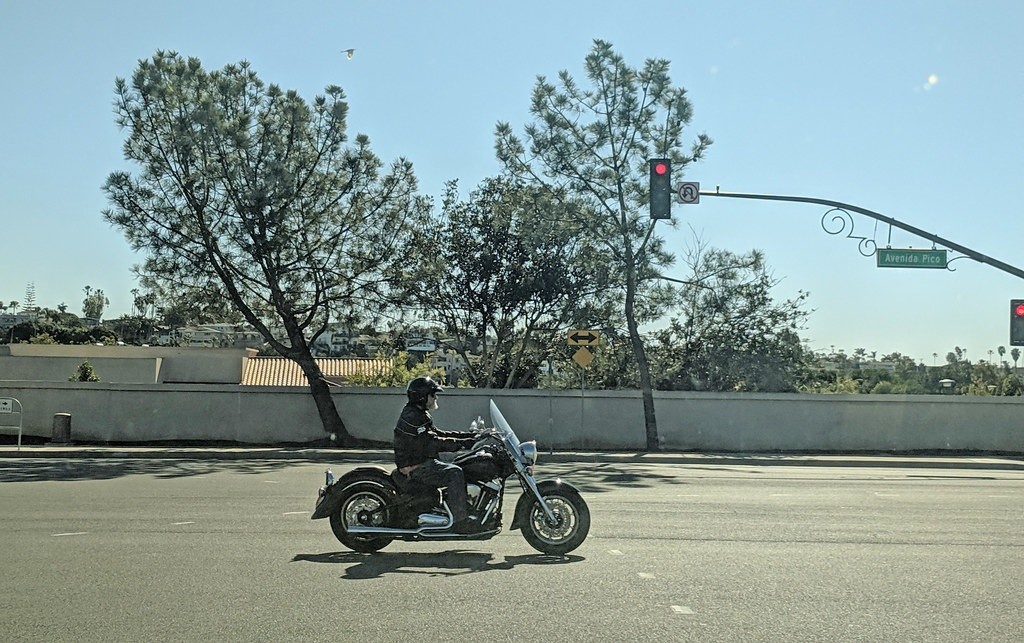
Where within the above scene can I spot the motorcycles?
[310,398,591,556]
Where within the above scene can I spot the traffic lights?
[1009,299,1024,347]
[649,157,671,219]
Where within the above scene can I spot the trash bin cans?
[51,413,72,443]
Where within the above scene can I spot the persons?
[393,377,480,533]
